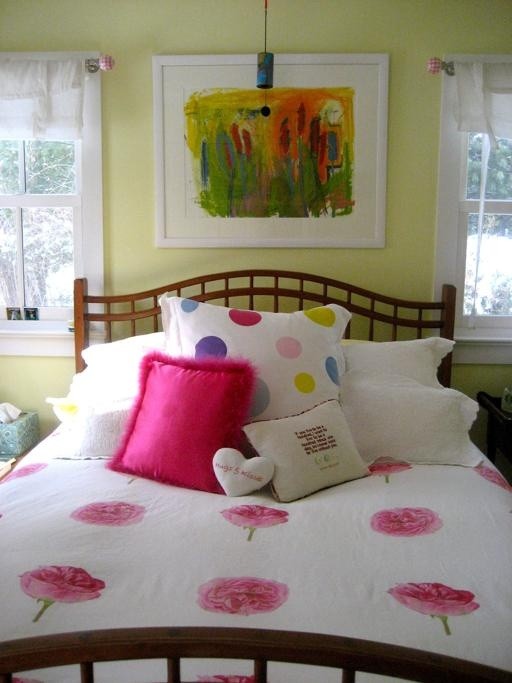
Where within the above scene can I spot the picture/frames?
[144,47,394,251]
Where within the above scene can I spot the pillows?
[105,348,256,495]
[339,338,455,390]
[159,294,351,420]
[243,400,372,503]
[339,374,483,467]
[51,331,165,461]
[213,448,274,497]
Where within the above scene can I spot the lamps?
[256,0,275,90]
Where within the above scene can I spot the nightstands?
[475,390,512,485]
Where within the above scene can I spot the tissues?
[0,402,39,456]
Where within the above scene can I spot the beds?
[0,257,512,682]
